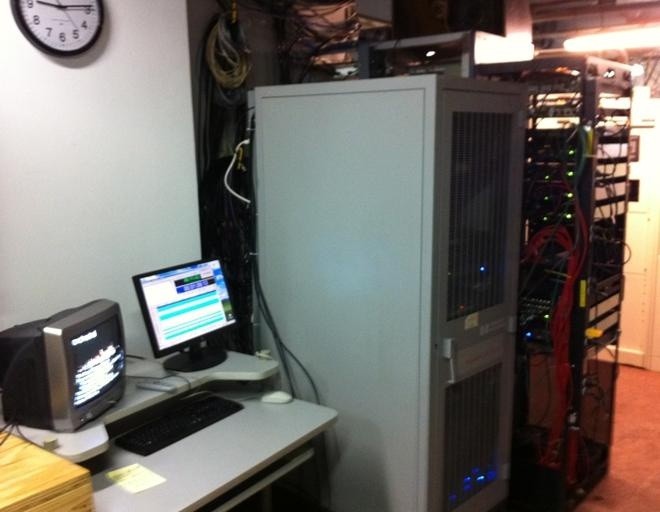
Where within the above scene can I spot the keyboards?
[115,396,244,455]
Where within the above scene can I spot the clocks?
[7,1,106,58]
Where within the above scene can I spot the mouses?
[262,390,292,403]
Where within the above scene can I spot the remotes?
[137,377,177,393]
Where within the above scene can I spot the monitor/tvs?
[0,298,125,433]
[133,255,241,372]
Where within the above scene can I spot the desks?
[0,345,340,512]
